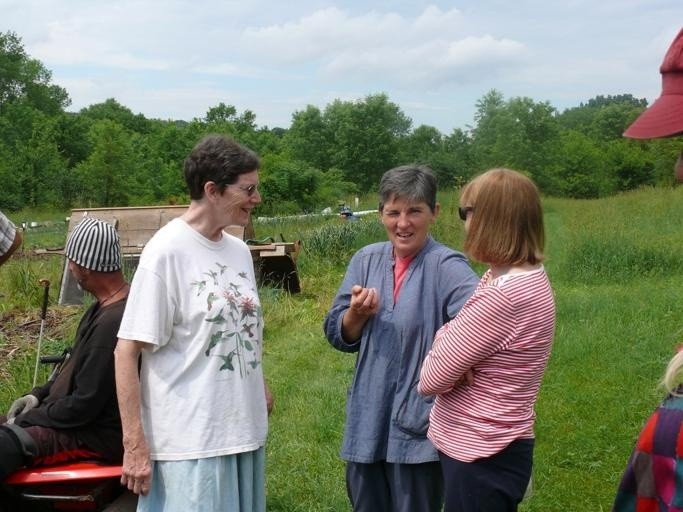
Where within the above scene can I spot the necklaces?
[98,282,127,304]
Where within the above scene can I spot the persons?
[419,169,556,511]
[0,208,23,273]
[1,216,156,512]
[325,166,477,510]
[607,27,683,511]
[114,133,276,511]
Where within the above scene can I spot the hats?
[622,28,683,139]
[65,218,122,272]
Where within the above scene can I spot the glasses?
[457,205,476,220]
[224,180,263,196]
